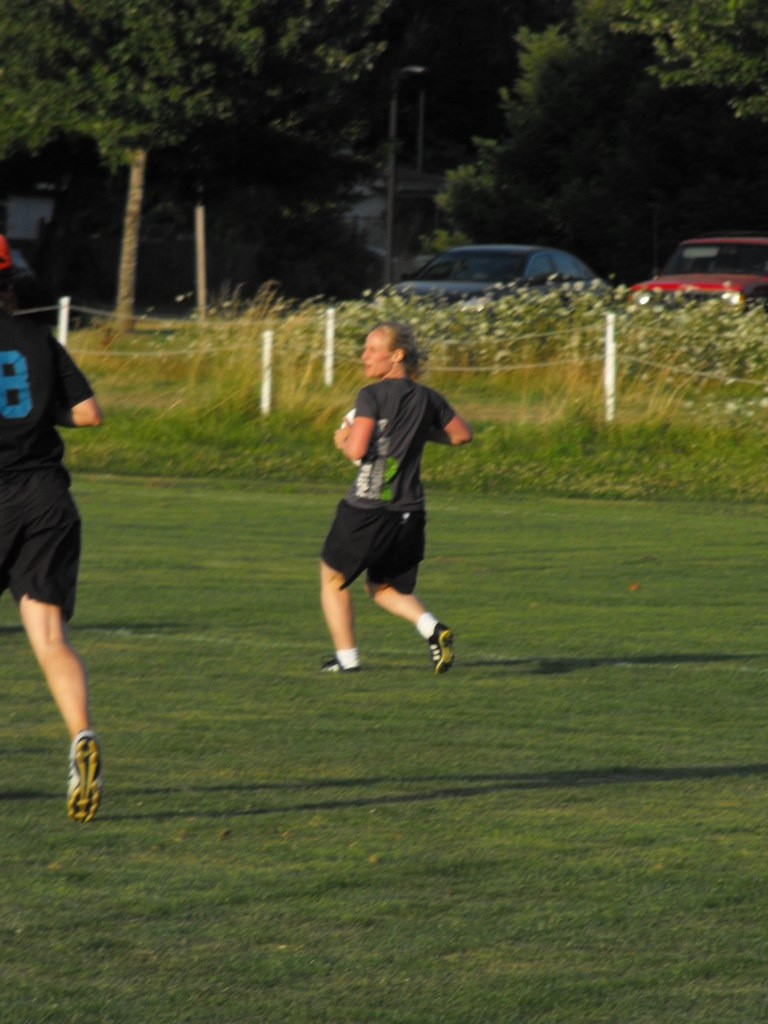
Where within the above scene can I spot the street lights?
[382,65,426,283]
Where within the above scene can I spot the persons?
[0,232,102,820]
[318,322,474,675]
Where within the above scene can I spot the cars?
[626,233,767,313]
[382,244,614,308]
[1,192,85,317]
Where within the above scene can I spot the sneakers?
[65,729,103,824]
[428,622,455,676]
[319,657,362,675]
